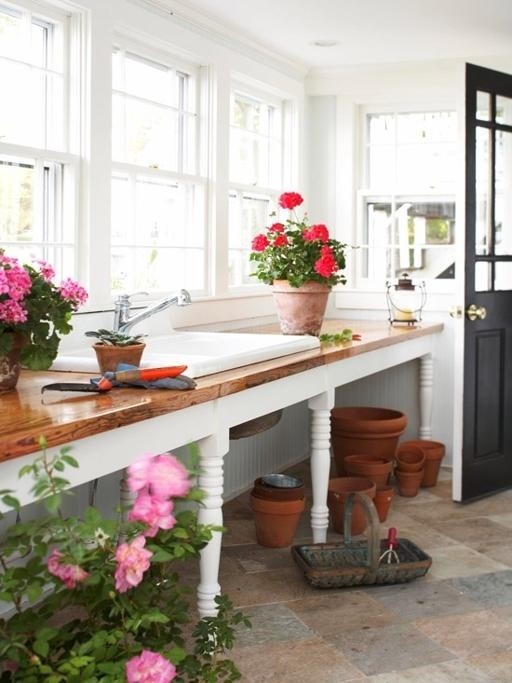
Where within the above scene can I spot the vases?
[1,333,24,394]
[250,405,446,548]
[271,277,331,337]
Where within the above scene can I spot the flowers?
[248,191,360,290]
[1,433,255,682]
[1,246,89,371]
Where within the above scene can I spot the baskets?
[293,490,432,589]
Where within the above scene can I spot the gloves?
[115,364,196,390]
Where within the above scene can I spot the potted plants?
[85,328,146,376]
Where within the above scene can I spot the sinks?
[55,328,321,382]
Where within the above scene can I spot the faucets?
[110,286,189,343]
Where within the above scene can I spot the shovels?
[39,365,189,394]
